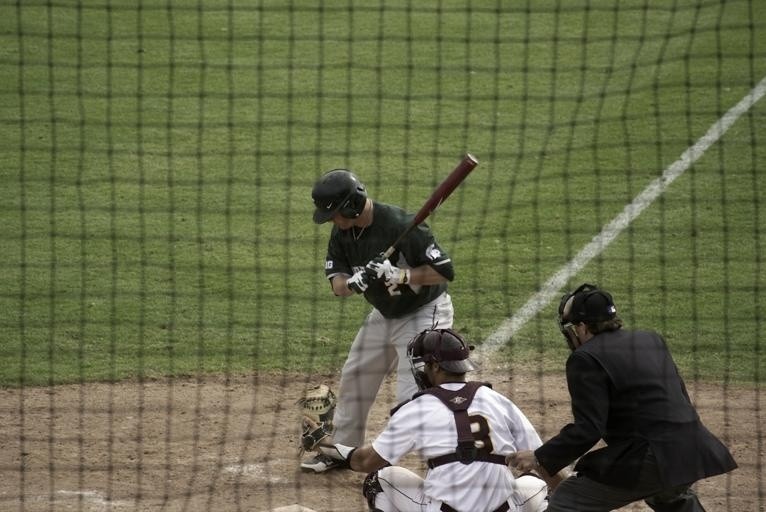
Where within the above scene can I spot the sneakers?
[298,455,344,472]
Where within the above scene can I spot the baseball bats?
[382,153,478,263]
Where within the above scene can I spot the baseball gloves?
[298,384,336,451]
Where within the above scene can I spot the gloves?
[344,270,368,294]
[366,256,397,285]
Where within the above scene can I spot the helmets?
[310,168,368,224]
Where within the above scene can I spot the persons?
[299,328,566,510]
[504,282,741,511]
[299,166,457,475]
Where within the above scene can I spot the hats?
[412,326,478,374]
[558,282,617,318]
